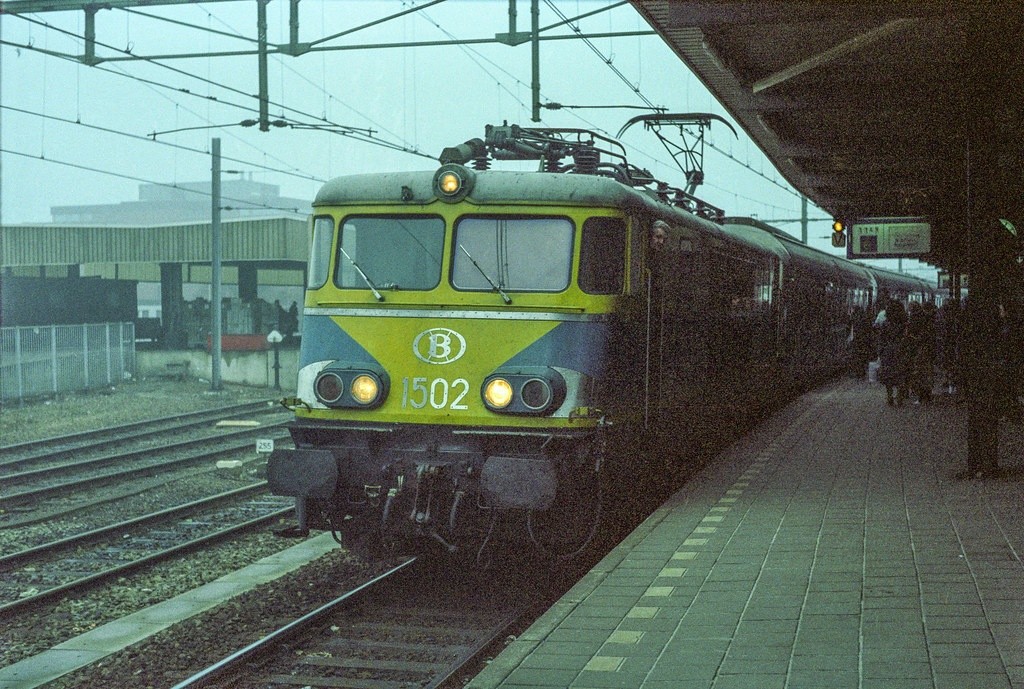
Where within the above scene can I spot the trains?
[271,122,969,572]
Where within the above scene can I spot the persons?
[650,219,672,253]
[876,299,1024,425]
